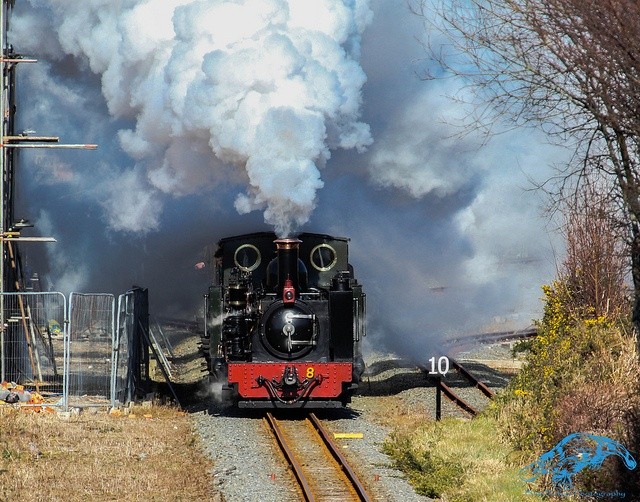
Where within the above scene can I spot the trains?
[197,232,365,412]
